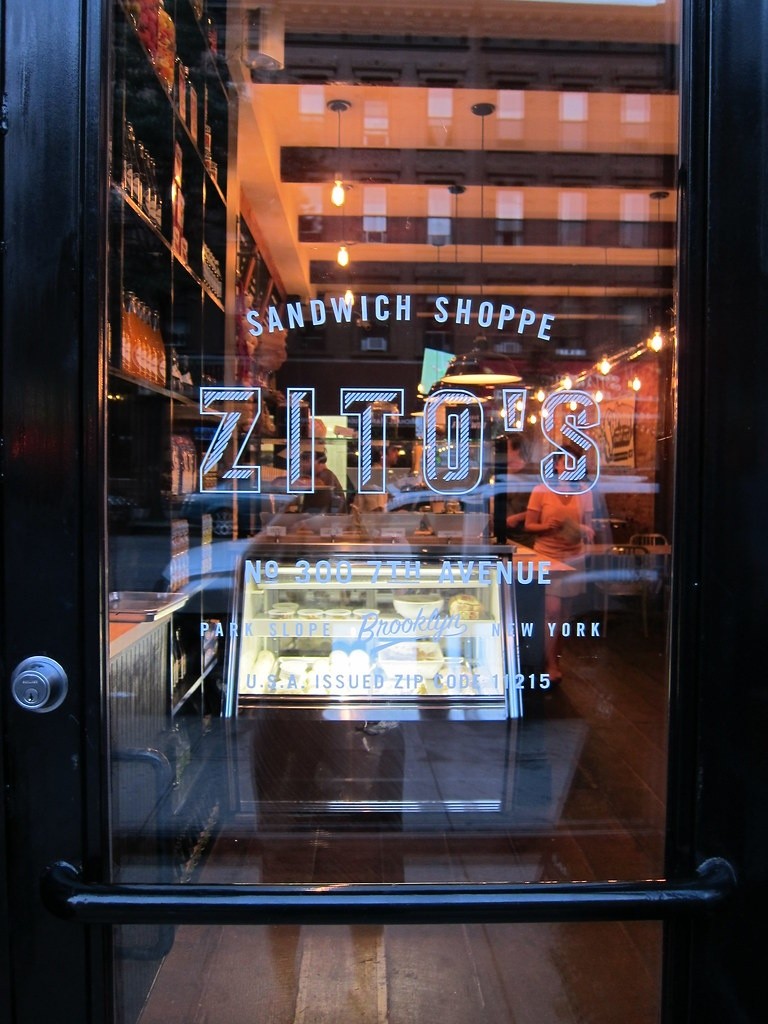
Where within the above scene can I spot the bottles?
[122,286,166,388]
[202,515,213,577]
[204,242,224,300]
[170,519,190,592]
[170,350,194,400]
[204,374,218,408]
[174,52,197,145]
[120,117,162,231]
[171,436,198,494]
[173,775,222,883]
[172,718,192,780]
[202,452,218,491]
[173,629,187,693]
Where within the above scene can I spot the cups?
[433,503,445,513]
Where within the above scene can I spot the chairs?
[630,533,668,547]
[592,545,652,637]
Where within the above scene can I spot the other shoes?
[540,667,562,682]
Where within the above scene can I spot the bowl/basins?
[279,662,309,683]
[393,594,444,619]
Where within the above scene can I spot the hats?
[303,443,326,453]
[375,444,402,452]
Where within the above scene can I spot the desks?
[583,542,672,629]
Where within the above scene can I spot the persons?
[297,451,346,517]
[487,433,597,684]
[353,443,399,512]
[237,468,406,1024]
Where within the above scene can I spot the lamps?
[600,248,610,375]
[571,401,577,409]
[543,408,548,417]
[563,265,572,391]
[531,416,536,424]
[439,102,523,385]
[595,389,603,402]
[516,421,524,429]
[344,263,355,307]
[422,184,491,405]
[499,409,508,418]
[650,192,670,352]
[537,386,545,402]
[516,400,524,411]
[632,374,642,392]
[337,184,352,266]
[327,100,351,206]
